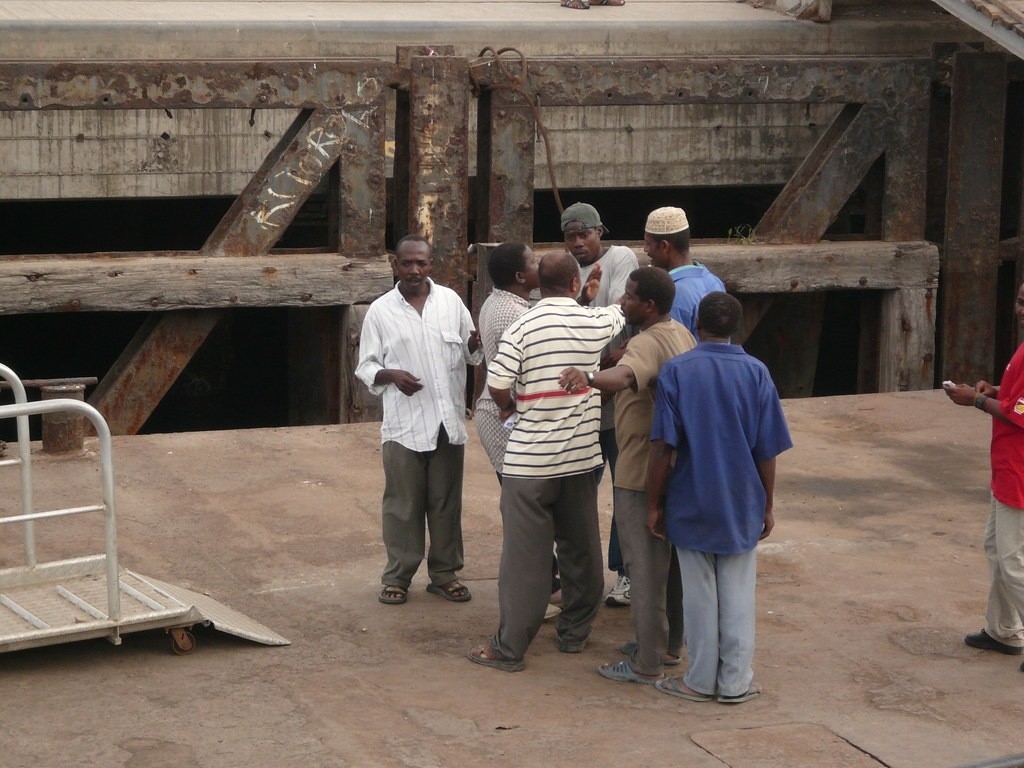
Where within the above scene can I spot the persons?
[464,250,651,672]
[356,236,486,605]
[558,265,697,685]
[472,202,731,619]
[645,291,793,702]
[942,282,1024,672]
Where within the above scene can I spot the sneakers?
[605,576,631,606]
[964,628,1023,655]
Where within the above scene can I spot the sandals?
[426,580,471,602]
[379,586,408,604]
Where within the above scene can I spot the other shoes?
[544,603,561,620]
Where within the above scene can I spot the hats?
[645,207,689,234]
[560,202,609,234]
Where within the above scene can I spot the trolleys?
[0,364,292,655]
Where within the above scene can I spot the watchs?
[584,371,596,386]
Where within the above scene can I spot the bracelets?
[975,393,989,411]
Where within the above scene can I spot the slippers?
[598,660,664,685]
[655,674,714,703]
[552,629,585,652]
[467,644,524,672]
[621,640,682,665]
[715,683,761,703]
